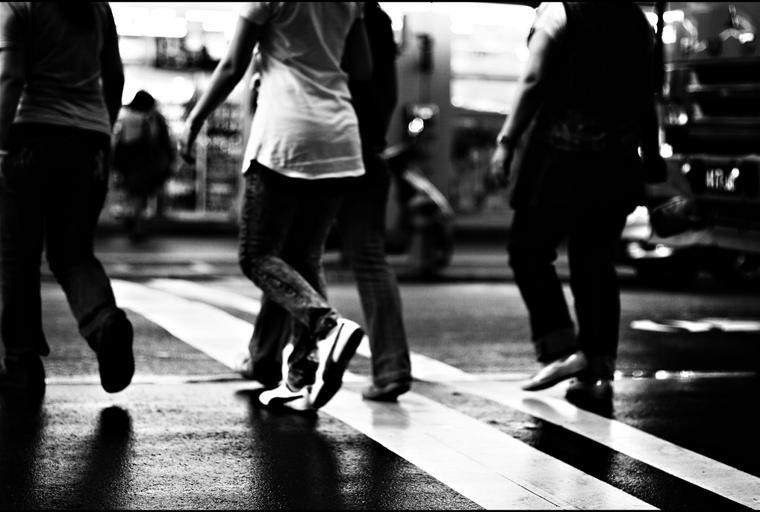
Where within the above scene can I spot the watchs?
[496,135,515,146]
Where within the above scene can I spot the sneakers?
[92,311,136,394]
[306,315,366,411]
[521,350,588,394]
[565,378,614,402]
[256,378,315,418]
[362,377,412,402]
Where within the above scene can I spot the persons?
[112,89,174,218]
[0,0,134,404]
[489,1,668,413]
[176,1,376,409]
[237,1,413,402]
